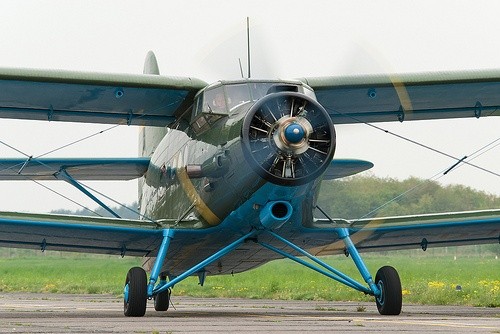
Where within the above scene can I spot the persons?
[209,93,231,118]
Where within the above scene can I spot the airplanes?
[0,48,500,316]
